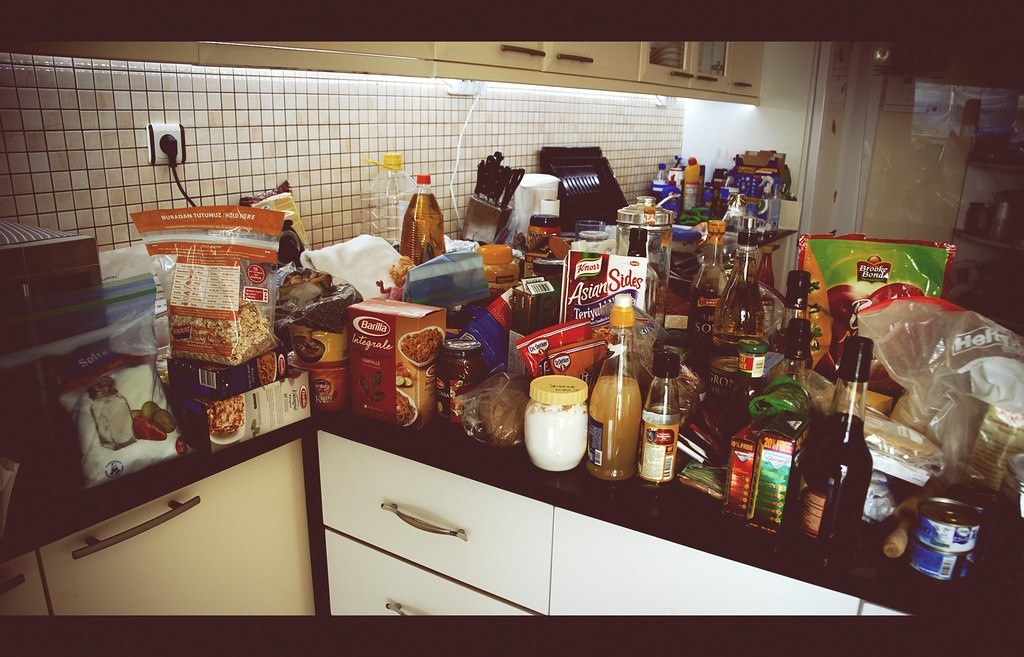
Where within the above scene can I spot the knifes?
[475,151,526,208]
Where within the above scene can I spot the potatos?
[130,401,176,433]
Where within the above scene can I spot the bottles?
[437,158,874,548]
[87,375,136,449]
[361,153,416,255]
[400,176,444,264]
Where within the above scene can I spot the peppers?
[133,416,167,441]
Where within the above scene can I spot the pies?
[199,395,244,435]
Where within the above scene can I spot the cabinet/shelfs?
[0,41,765,106]
[950,132,1024,328]
[0,432,909,615]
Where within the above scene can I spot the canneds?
[909,484,984,582]
[999,453,1024,519]
[528,214,561,248]
[524,375,589,471]
[577,231,609,242]
[435,338,484,425]
[476,244,519,295]
[710,356,738,409]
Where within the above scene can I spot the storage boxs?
[724,409,810,535]
[866,390,893,418]
[346,297,447,430]
[735,150,786,231]
[507,276,562,374]
[167,350,312,454]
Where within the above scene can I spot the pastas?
[402,327,442,363]
[396,391,414,425]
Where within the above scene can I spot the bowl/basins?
[399,327,444,366]
[285,322,349,412]
[210,410,246,445]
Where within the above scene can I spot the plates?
[395,387,419,427]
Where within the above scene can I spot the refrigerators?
[682,41,853,316]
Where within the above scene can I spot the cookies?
[359,371,385,402]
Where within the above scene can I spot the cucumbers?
[396,376,413,387]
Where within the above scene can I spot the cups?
[574,220,606,241]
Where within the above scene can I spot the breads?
[478,389,528,447]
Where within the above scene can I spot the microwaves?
[0,222,111,471]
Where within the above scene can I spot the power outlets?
[146,123,186,165]
